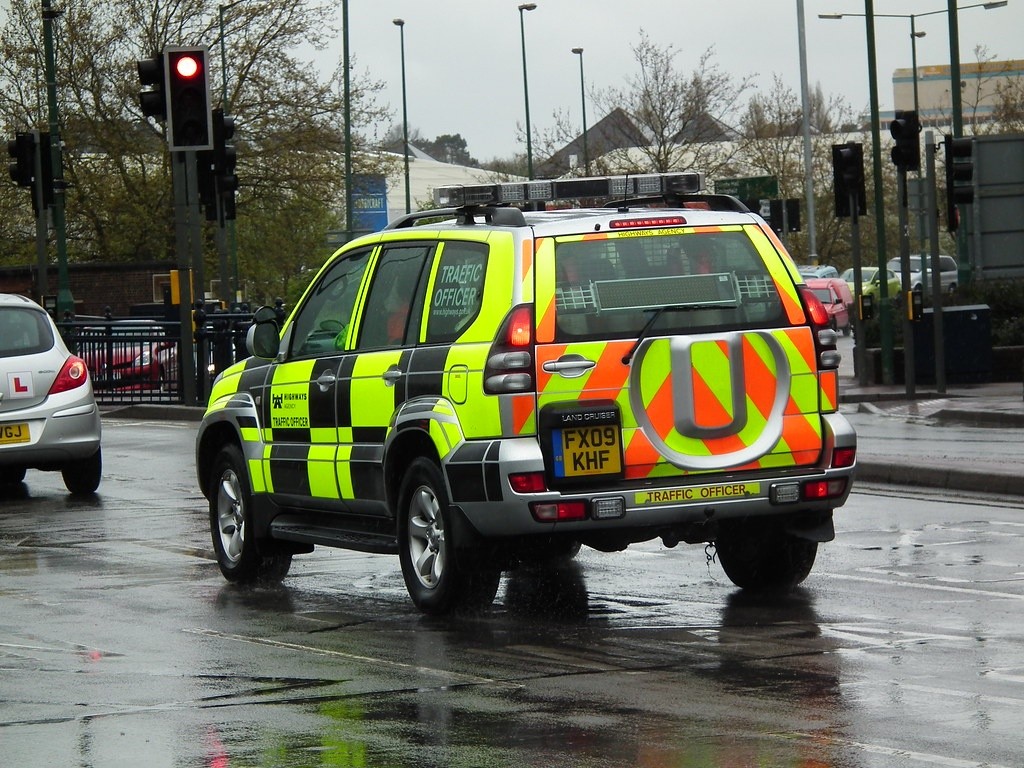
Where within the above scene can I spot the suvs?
[195,197,858,618]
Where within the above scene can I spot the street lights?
[818,0,1008,308]
[393,19,411,216]
[519,3,537,181]
[572,47,589,177]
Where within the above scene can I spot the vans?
[805,278,854,336]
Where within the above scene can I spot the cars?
[886,255,959,295]
[840,266,900,306]
[77,319,196,393]
[0,293,102,494]
[797,265,838,278]
[300,331,345,355]
[159,324,237,391]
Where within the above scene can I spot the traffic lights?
[8,132,36,186]
[197,108,238,221]
[945,134,975,230]
[163,46,213,151]
[890,110,920,172]
[137,52,167,122]
